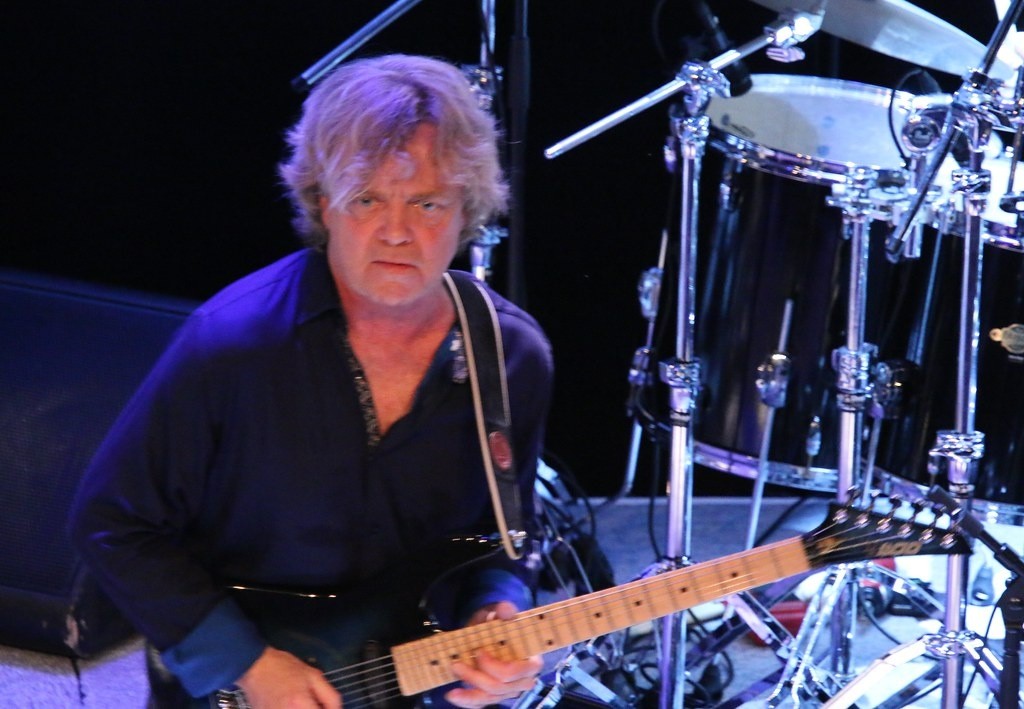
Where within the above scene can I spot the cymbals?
[750,0,989,84]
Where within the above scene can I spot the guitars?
[144,485,976,709]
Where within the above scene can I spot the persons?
[66,54,553,708]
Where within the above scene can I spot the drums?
[635,74,1024,527]
